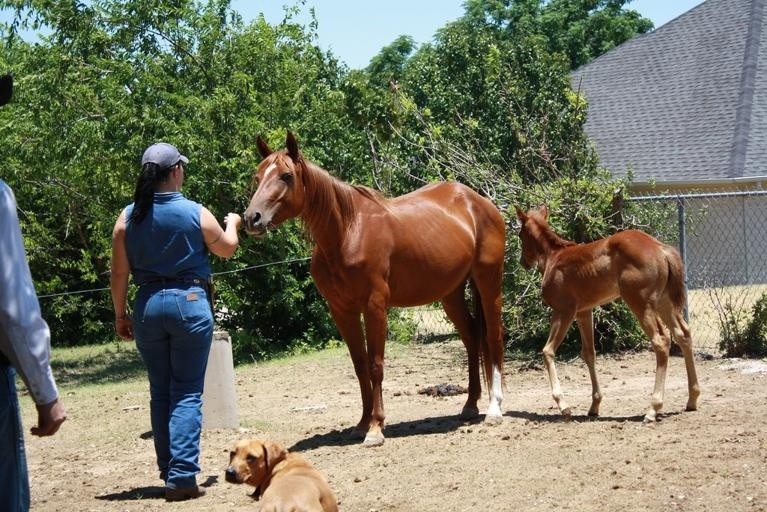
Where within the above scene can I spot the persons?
[109,142,243,499]
[0,71,68,512]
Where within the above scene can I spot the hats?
[143,142,189,168]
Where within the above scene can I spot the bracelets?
[115,314,126,320]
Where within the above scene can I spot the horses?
[239,128,507,449]
[513,202,701,425]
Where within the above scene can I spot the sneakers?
[165,481,206,501]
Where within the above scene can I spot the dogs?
[224,436,341,512]
